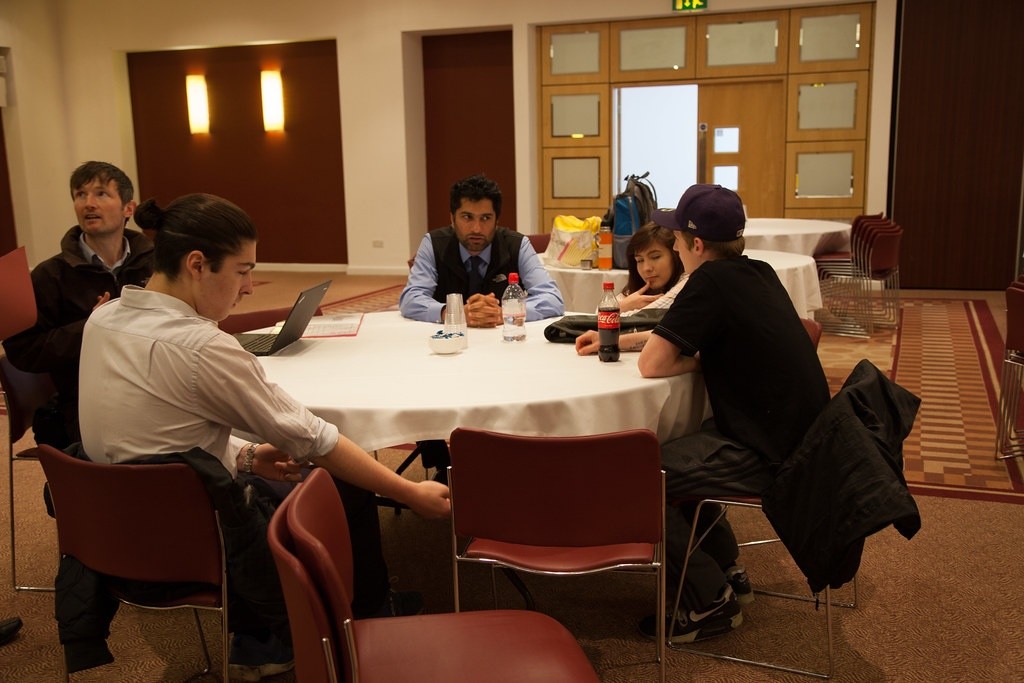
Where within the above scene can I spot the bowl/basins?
[427,335,466,354]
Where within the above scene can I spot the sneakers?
[727,568,754,604]
[222,631,295,682]
[639,583,744,643]
[368,575,425,617]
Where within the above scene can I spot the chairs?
[663,360,877,678]
[811,214,903,338]
[1,350,62,592]
[35,445,260,683]
[994,282,1024,459]
[265,468,599,682]
[448,426,669,663]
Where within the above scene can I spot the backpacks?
[614,172,656,268]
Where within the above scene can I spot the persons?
[576,184,831,642]
[78,193,451,683]
[596,221,691,316]
[399,175,564,485]
[2,161,155,442]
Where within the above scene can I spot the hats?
[652,183,746,241]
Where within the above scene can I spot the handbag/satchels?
[543,214,602,269]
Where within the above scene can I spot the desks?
[744,218,853,258]
[230,311,710,514]
[537,249,822,322]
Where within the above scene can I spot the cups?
[443,293,467,341]
[580,260,594,270]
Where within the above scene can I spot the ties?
[468,256,482,290]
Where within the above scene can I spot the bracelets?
[243,443,259,472]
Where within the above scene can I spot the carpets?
[319,284,1024,505]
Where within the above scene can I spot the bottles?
[502,273,525,341]
[598,281,621,362]
[598,227,613,272]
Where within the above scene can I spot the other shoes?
[0,616,23,643]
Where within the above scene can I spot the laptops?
[232,280,333,356]
